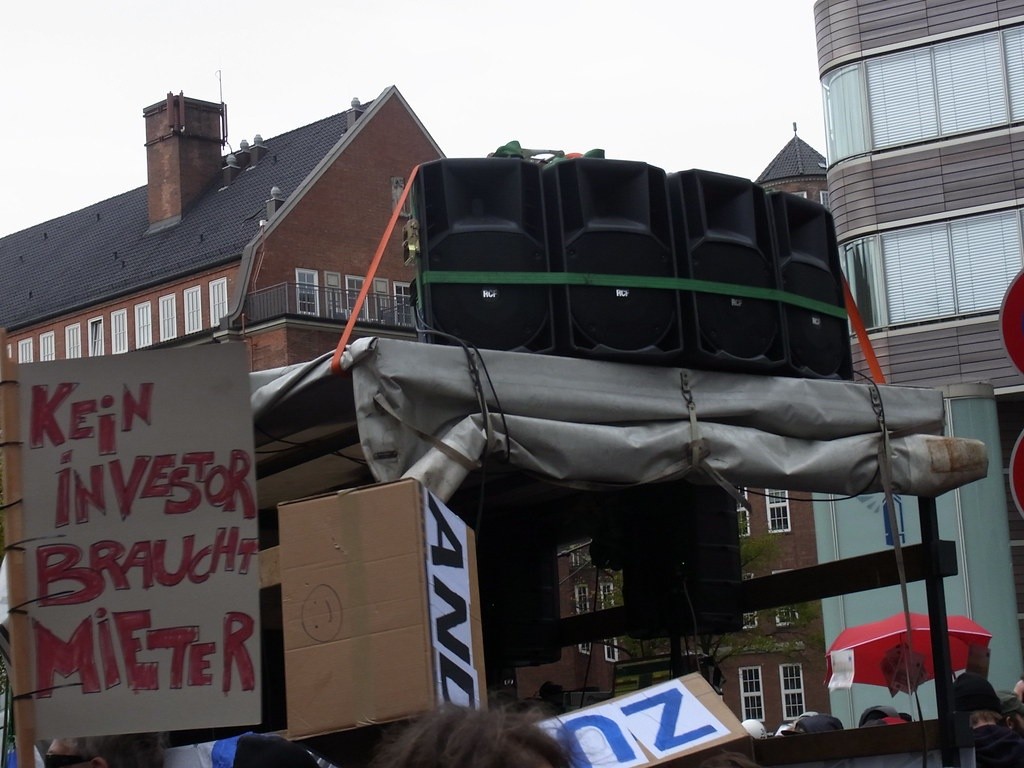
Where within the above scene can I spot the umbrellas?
[824,611,991,721]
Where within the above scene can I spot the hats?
[860,716,909,728]
[995,688,1024,716]
[951,673,1002,715]
[781,724,807,736]
[741,719,767,739]
[232,734,320,768]
[799,711,819,717]
[211,736,240,768]
[858,704,899,728]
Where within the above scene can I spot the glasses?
[44,754,113,768]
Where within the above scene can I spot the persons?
[742,705,915,739]
[954,672,1024,768]
[44,731,169,768]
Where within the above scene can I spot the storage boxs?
[278,476,488,741]
